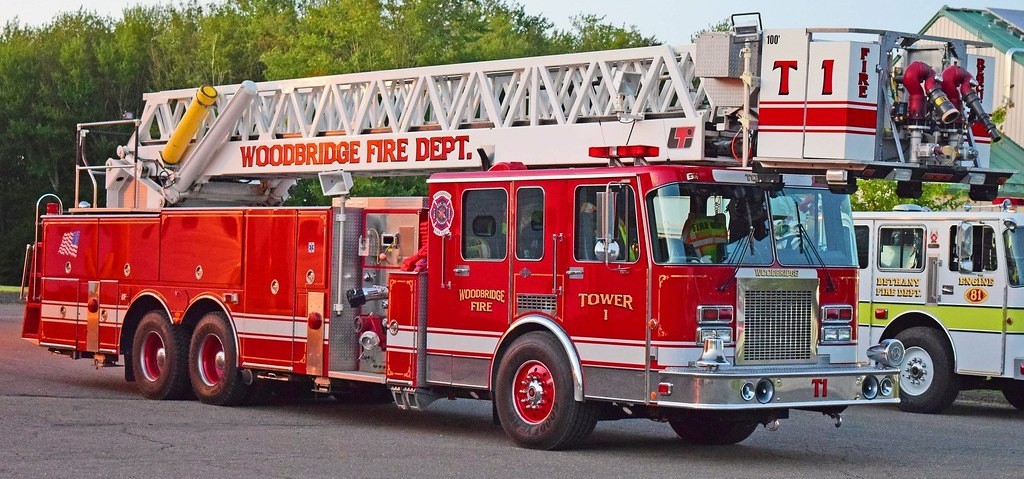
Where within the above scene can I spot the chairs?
[637,238,699,264]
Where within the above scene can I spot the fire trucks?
[819,197,1024,415]
[20,12,1020,451]
